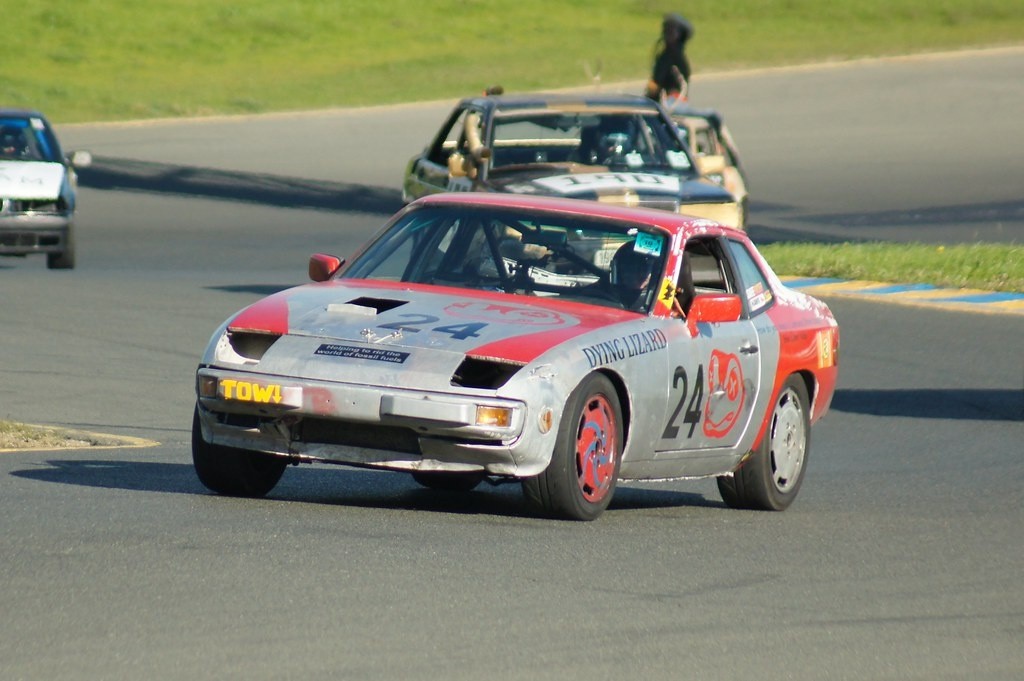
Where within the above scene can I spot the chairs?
[593,240,697,319]
[578,126,635,165]
[477,236,524,281]
[0,126,29,157]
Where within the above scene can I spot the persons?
[594,241,681,317]
[642,15,697,106]
[594,127,632,167]
[0,131,24,158]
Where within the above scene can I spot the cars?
[401,91,750,265]
[0,110,92,268]
[191,192,840,520]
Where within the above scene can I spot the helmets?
[612,240,657,285]
[595,120,636,154]
[0,125,27,154]
